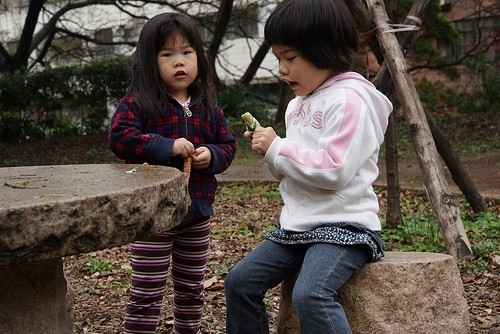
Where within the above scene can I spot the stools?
[275,250,471,334]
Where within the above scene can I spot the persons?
[107,12,237,334]
[222,0,394,333]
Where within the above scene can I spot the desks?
[0,163,190,334]
